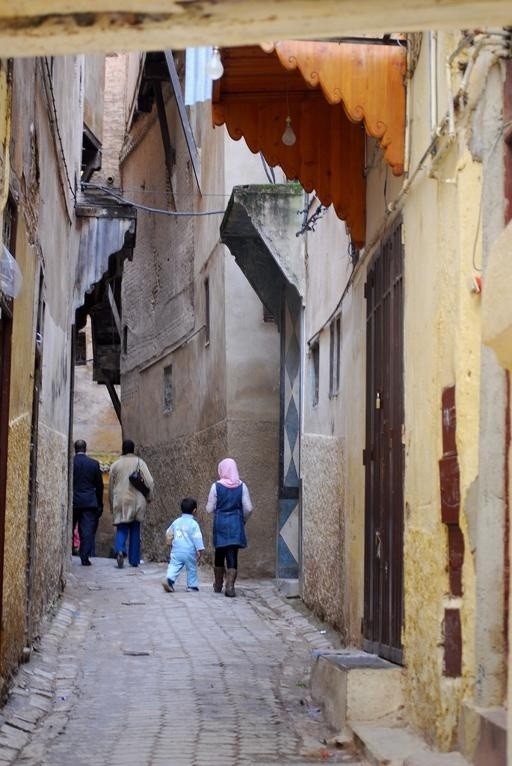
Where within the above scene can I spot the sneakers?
[187,586,198,591]
[163,578,173,591]
[116,551,124,568]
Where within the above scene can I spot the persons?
[206,457,254,599]
[160,497,205,594]
[107,438,155,570]
[73,438,104,567]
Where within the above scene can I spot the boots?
[213,567,225,592]
[225,568,236,597]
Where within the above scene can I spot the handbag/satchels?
[130,471,150,497]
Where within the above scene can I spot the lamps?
[206,47,224,79]
[280,95,297,146]
